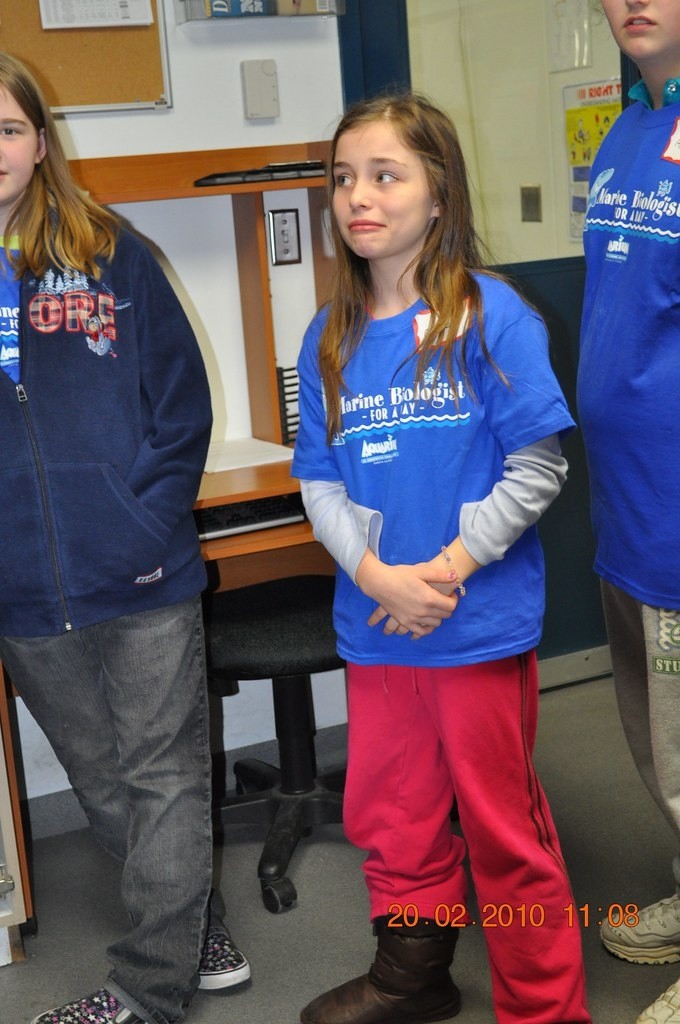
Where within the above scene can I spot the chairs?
[203,572,348,917]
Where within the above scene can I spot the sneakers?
[599,891,680,965]
[636,977,680,1024]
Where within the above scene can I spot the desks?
[0,459,316,935]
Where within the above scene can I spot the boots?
[300,917,462,1024]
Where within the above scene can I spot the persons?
[575,0,680,1024]
[290,95,592,1024]
[0,53,253,1024]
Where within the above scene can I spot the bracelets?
[441,546,468,597]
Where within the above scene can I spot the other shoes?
[31,990,147,1024]
[198,933,251,990]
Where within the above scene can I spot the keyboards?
[192,495,305,541]
